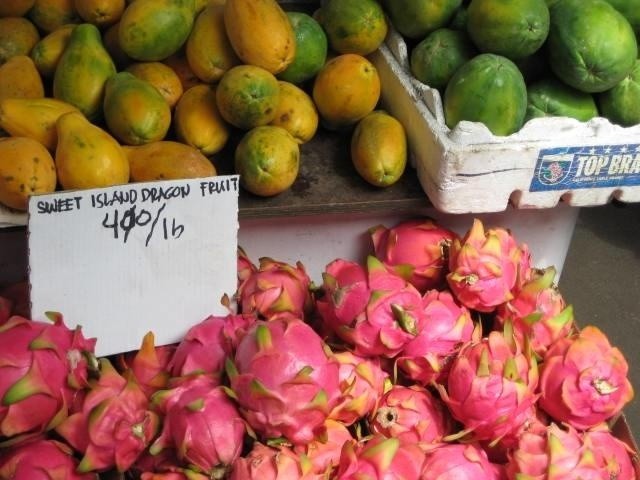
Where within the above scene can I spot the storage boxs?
[369,11,639,215]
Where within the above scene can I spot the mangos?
[0,1,640,211]
